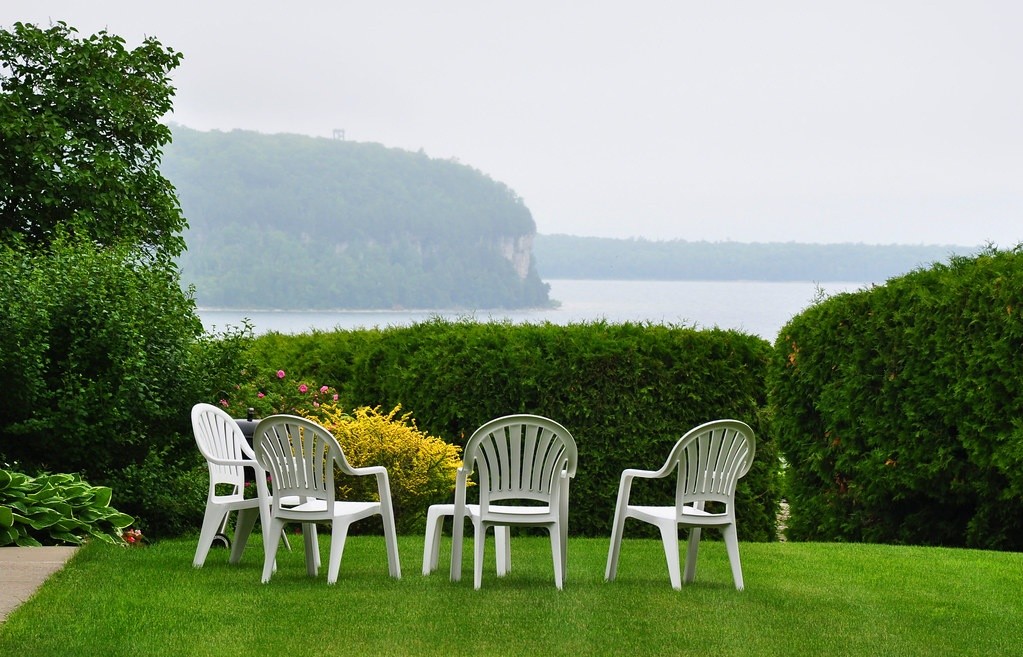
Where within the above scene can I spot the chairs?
[190,402,322,575]
[603,419,756,590]
[251,414,401,586]
[422,413,579,591]
